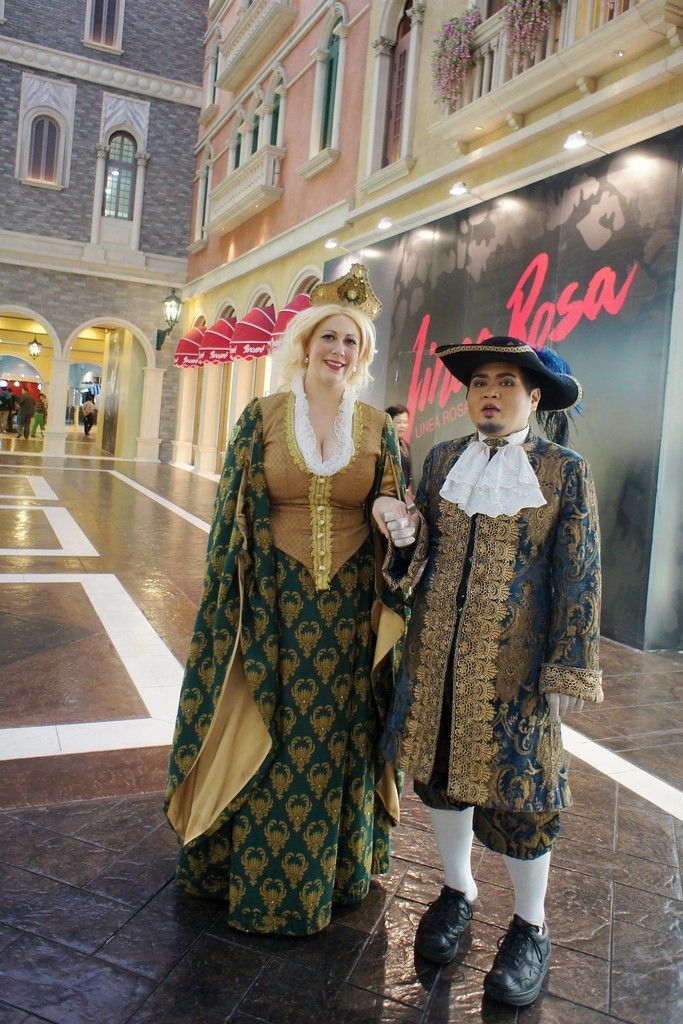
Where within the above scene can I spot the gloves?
[384,502,416,547]
[545,691,584,720]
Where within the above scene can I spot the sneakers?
[414,885,477,963]
[484,914,551,1006]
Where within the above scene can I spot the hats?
[435,336,584,447]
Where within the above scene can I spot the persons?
[31,393,48,437]
[161,307,409,936]
[375,337,604,1008]
[82,395,95,436]
[0,387,19,433]
[386,405,414,502]
[16,388,35,439]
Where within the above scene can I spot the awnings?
[229,305,275,362]
[173,326,206,369]
[270,295,312,347]
[195,317,237,365]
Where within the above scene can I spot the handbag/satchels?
[406,484,415,504]
